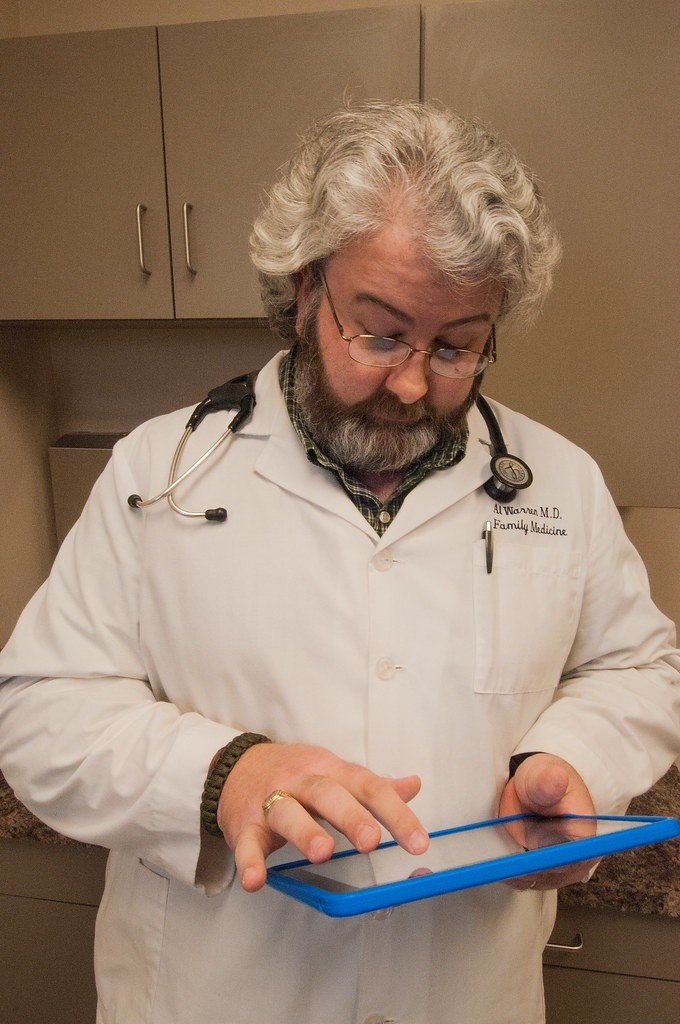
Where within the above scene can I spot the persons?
[0,101,680,1024]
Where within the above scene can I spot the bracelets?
[199,732,272,838]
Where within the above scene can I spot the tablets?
[264,809,680,918]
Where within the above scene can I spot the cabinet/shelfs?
[0,0,680,1024]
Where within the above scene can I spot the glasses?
[317,266,497,381]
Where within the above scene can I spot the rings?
[262,790,288,815]
